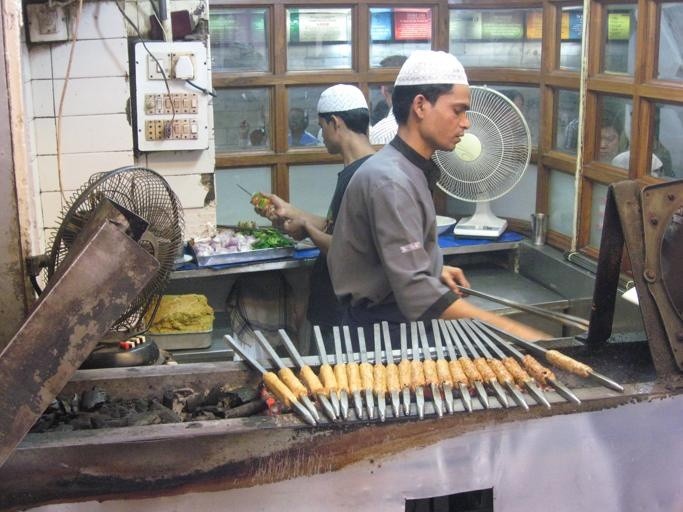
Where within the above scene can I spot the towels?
[228,271,297,362]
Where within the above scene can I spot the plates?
[169,255,192,271]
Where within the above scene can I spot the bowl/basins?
[435,213,456,236]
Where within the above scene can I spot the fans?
[28,162,183,370]
[429,87,532,239]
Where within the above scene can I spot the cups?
[529,213,549,247]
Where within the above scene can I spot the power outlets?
[170,52,195,80]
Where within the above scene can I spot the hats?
[317,83,368,113]
[394,49,467,85]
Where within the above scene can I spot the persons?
[328,50,558,342]
[241,54,411,146]
[611,140,663,179]
[253,84,380,355]
[597,108,622,167]
[629,105,672,177]
[557,94,579,153]
[489,89,524,126]
